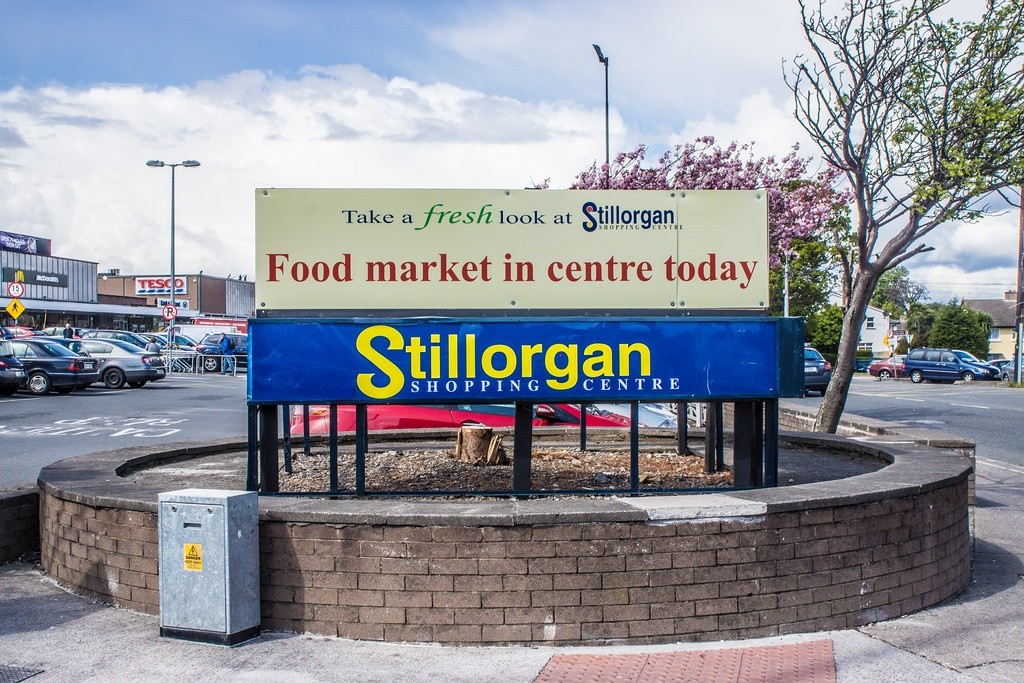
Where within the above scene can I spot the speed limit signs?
[8,282,24,298]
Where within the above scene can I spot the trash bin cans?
[855,360,871,372]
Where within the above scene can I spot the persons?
[144,338,160,353]
[63,323,74,338]
[218,333,234,376]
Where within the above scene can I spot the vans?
[901,348,1001,383]
[162,324,237,343]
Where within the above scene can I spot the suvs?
[195,333,248,371]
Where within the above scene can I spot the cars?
[952,350,981,362]
[288,403,691,435]
[804,347,832,396]
[985,355,1024,383]
[0,326,198,395]
[869,354,911,381]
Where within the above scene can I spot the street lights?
[591,43,609,189]
[145,159,202,324]
